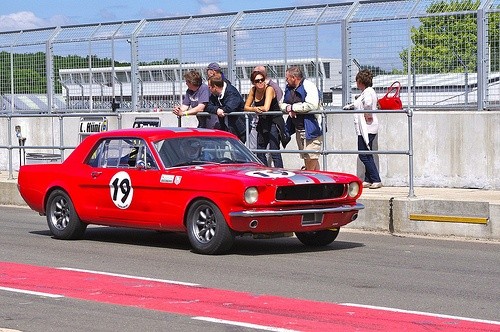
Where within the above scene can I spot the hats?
[206,62,221,71]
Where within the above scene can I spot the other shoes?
[369,182,383,189]
[362,181,371,188]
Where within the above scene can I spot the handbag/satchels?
[378,80,404,110]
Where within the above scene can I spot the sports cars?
[16,127,365,256]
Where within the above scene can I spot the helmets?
[179,136,200,159]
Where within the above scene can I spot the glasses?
[255,77,265,83]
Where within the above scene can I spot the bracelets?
[185,111,188,116]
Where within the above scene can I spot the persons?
[244,71,285,168]
[253,66,283,103]
[278,66,324,171]
[204,75,253,162]
[173,71,228,161]
[206,62,232,86]
[353,69,383,189]
